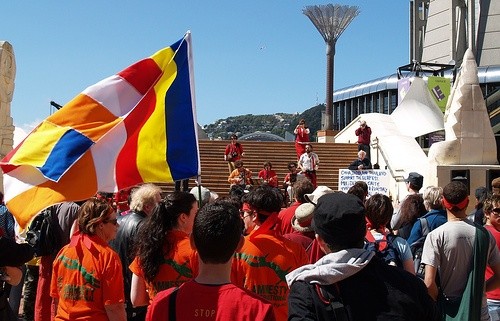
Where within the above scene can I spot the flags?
[0,31,202,236]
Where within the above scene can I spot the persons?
[0,119,500,321]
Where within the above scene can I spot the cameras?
[361,124,365,127]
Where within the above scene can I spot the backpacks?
[25,204,61,257]
[363,233,402,270]
[409,217,430,280]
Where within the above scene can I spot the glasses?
[238,209,251,218]
[491,208,500,215]
[104,219,117,225]
[236,166,243,168]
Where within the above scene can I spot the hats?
[290,203,316,231]
[404,172,424,186]
[304,185,335,204]
[0,239,34,267]
[313,190,365,242]
